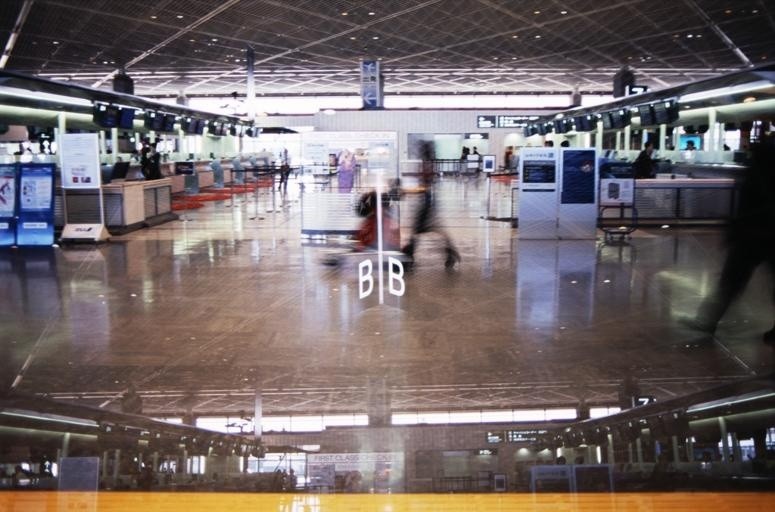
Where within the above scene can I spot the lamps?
[112,50,135,95]
[613,49,634,98]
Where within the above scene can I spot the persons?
[336,149,356,193]
[397,139,461,270]
[279,149,291,189]
[561,140,569,147]
[544,141,553,146]
[462,146,480,159]
[632,142,656,179]
[686,140,696,150]
[504,147,514,172]
[677,118,775,343]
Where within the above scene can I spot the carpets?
[169,175,272,211]
